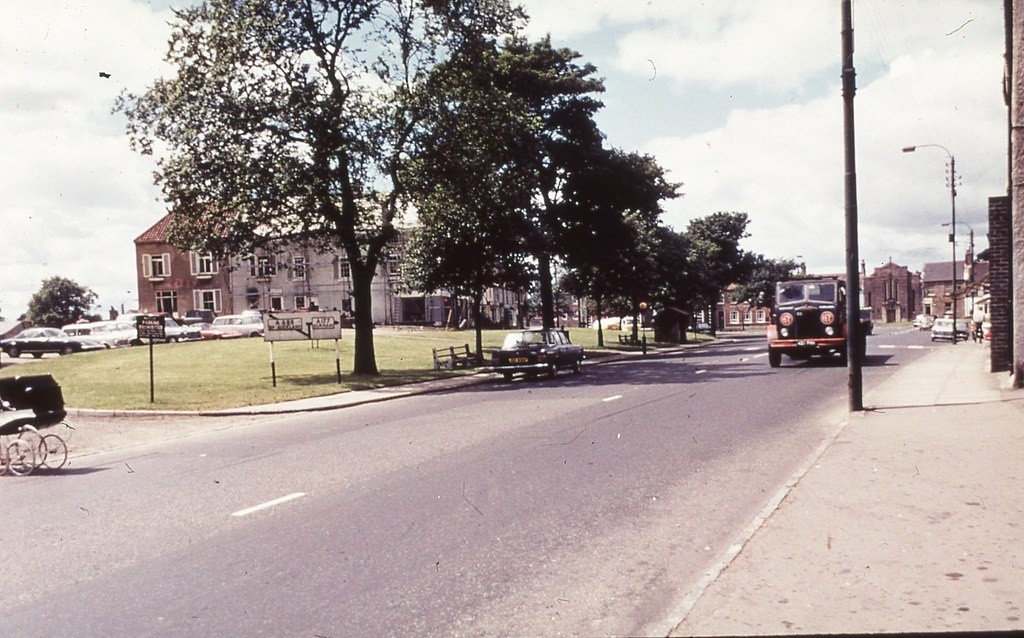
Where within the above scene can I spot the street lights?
[942,222,974,316]
[639,302,648,355]
[902,144,960,346]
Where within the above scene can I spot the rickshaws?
[0,374,76,478]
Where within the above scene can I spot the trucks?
[766,276,874,365]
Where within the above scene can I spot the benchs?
[618,334,641,346]
[430,343,480,369]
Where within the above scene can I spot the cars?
[492,330,585,384]
[58,307,264,351]
[912,313,969,342]
[697,323,712,335]
[0,327,82,357]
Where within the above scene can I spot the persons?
[972,307,985,343]
